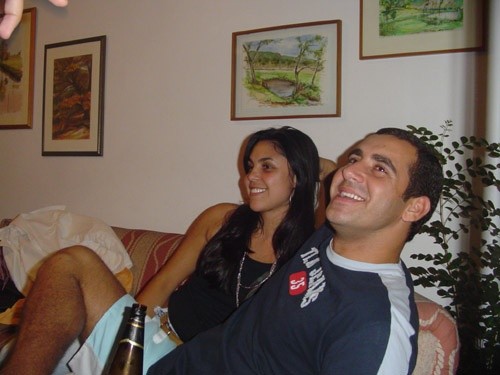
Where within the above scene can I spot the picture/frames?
[231,19,342,120]
[0,7,36,129]
[42,35,106,156]
[359,0,486,60]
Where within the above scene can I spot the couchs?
[0,218,461,375]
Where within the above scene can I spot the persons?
[0,0,68,39]
[136,125,320,347]
[0,128,444,375]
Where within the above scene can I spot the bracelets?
[160,321,172,335]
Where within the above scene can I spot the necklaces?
[235,251,278,307]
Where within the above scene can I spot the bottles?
[107,304,147,375]
